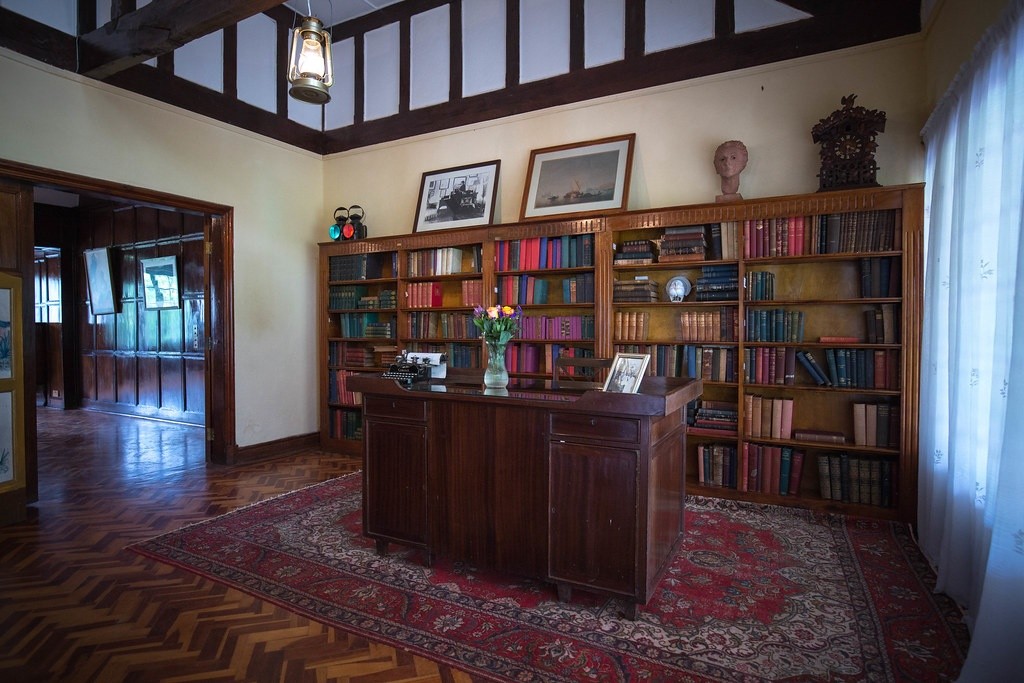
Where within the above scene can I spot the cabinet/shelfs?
[343,371,705,622]
[316,182,925,522]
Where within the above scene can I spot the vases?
[483,344,510,387]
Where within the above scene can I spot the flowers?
[471,303,521,342]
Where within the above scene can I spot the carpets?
[123,468,971,683]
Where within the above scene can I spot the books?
[328,253,397,442]
[406,245,483,369]
[743,212,902,508]
[613,222,738,491]
[494,235,595,376]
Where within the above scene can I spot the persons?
[459,180,465,191]
[713,139,749,202]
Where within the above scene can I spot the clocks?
[810,94,888,193]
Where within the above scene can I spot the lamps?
[285,0,333,105]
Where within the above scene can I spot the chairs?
[551,356,613,393]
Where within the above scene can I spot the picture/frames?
[83,247,116,316]
[140,254,180,310]
[603,352,652,393]
[517,133,636,222]
[0,271,26,494]
[412,158,501,234]
